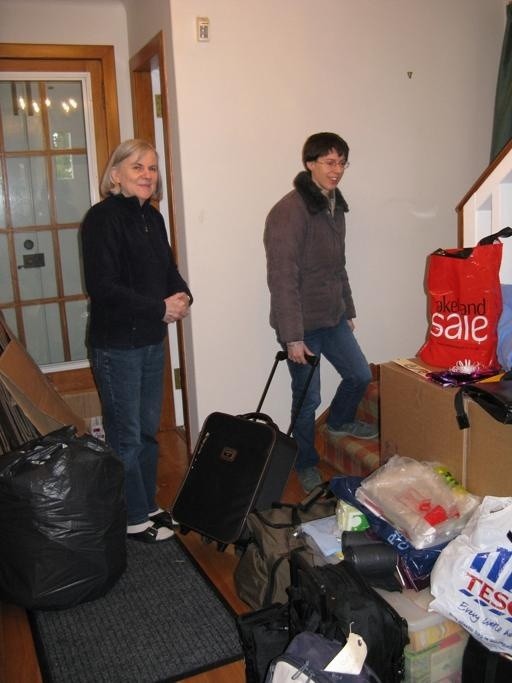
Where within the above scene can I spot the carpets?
[25,531,243,683]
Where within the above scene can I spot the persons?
[263,132,379,495]
[79,139,194,545]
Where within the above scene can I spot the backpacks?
[242,555,411,683]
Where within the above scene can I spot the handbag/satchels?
[416,226,512,373]
[234,488,326,611]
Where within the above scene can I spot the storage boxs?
[374,355,510,500]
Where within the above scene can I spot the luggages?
[169,351,320,557]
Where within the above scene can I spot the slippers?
[150,508,178,528]
[127,522,175,545]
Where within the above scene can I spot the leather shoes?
[298,465,323,495]
[326,418,379,441]
